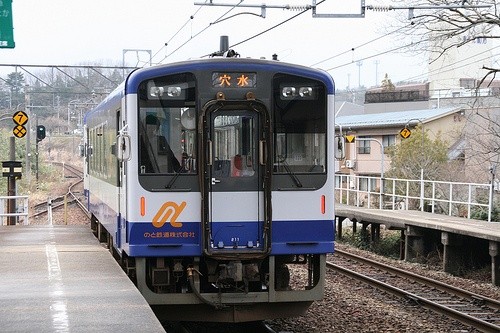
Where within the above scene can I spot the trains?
[79,43,339,320]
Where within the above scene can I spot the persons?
[141,115,184,174]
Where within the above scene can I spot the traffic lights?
[37,125,46,139]
